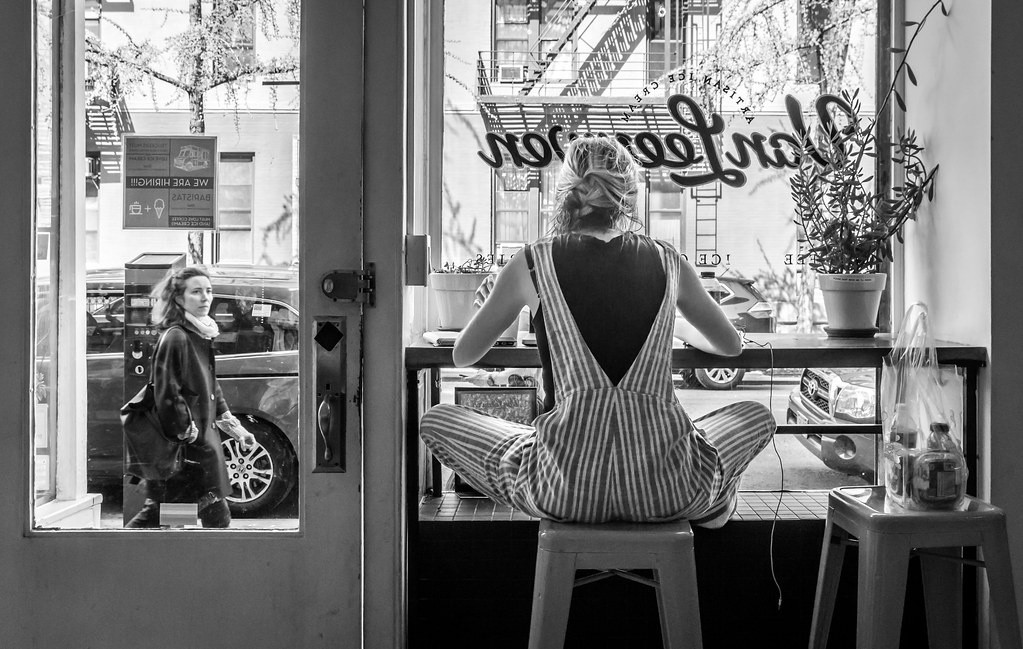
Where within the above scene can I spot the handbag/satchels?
[120,381,188,482]
[881,301,969,510]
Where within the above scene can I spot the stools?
[525,518,705,649]
[809,485,1021,649]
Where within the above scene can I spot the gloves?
[177,421,198,444]
[214,411,256,452]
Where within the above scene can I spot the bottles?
[889,399,965,510]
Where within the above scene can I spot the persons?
[124,269,242,529]
[420,138,777,529]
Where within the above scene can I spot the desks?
[407,330,986,521]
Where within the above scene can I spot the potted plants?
[790,0,955,338]
[430,254,498,332]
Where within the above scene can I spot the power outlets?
[408,234,432,288]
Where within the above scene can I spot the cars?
[35,251,883,518]
